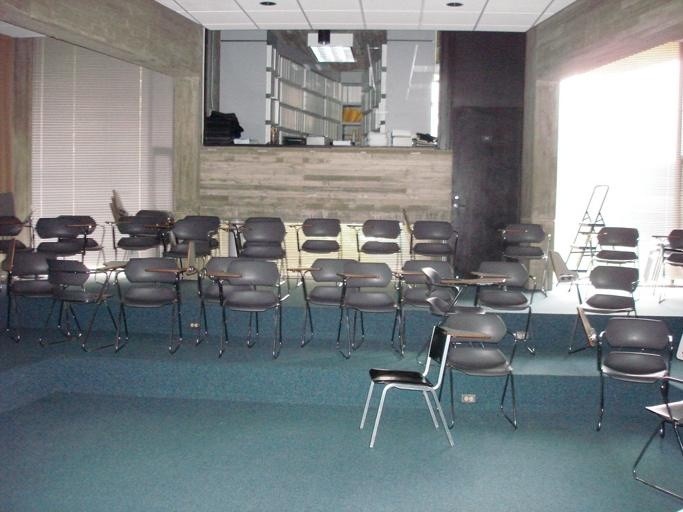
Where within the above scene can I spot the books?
[234,43,414,146]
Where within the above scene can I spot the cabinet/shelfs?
[219,29,438,145]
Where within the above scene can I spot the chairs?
[632,340,681,502]
[596,317,673,437]
[0,188,682,361]
[360,324,454,449]
[435,313,518,429]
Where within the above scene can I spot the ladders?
[556,185,615,293]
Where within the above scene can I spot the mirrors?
[201,29,443,148]
